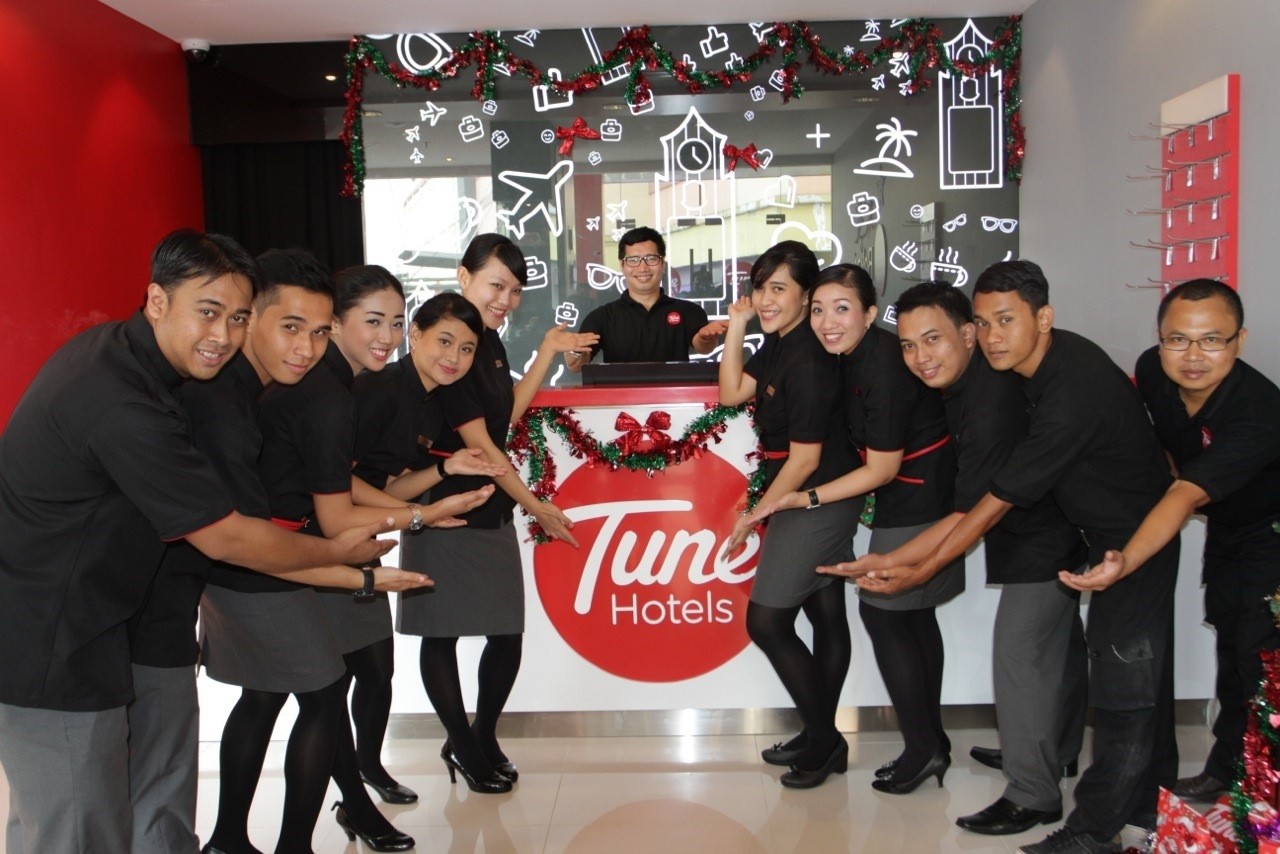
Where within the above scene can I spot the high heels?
[330,801,415,852]
[355,753,418,803]
[780,739,848,788]
[876,731,952,777]
[873,748,951,795]
[201,843,225,854]
[440,740,512,794]
[762,727,836,764]
[471,725,519,782]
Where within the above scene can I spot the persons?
[389,234,603,793]
[711,241,1179,854]
[122,235,506,854]
[0,227,396,854]
[1057,278,1280,806]
[562,225,727,372]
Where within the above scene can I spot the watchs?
[353,565,377,600]
[406,505,424,531]
[805,488,821,510]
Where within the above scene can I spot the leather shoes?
[956,798,1062,836]
[1016,826,1123,854]
[1171,773,1231,802]
[971,744,1078,778]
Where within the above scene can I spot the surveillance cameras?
[180,39,210,62]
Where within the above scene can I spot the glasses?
[622,253,663,267]
[1160,325,1244,352]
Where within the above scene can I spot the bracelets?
[437,459,451,479]
